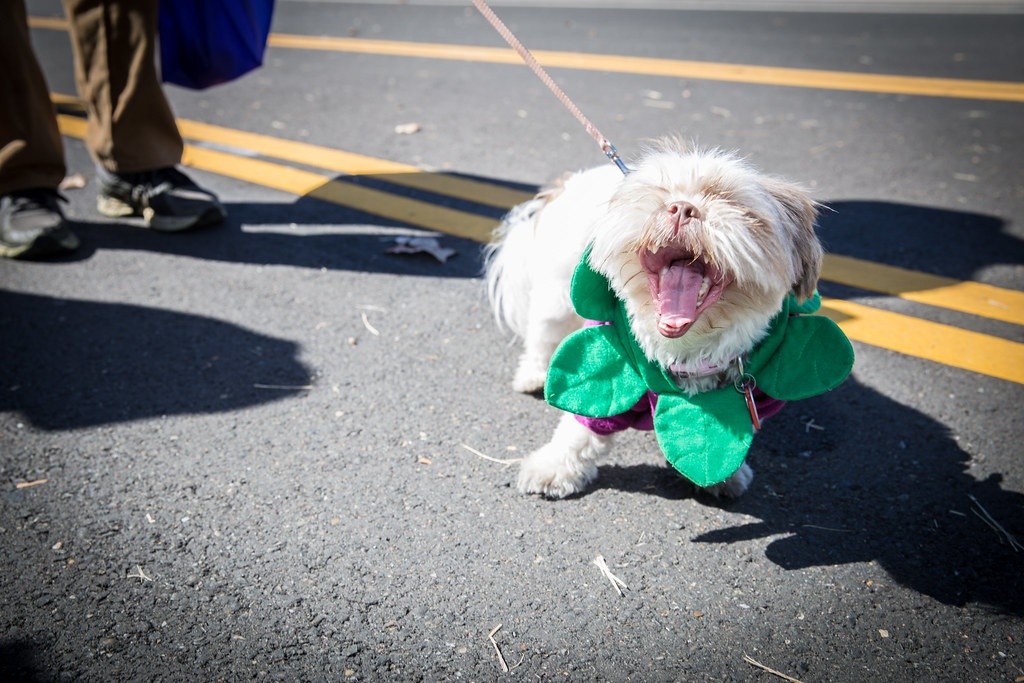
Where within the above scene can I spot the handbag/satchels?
[151,0,276,91]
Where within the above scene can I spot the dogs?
[480,131,840,500]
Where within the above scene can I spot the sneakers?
[95,165,227,235]
[0,189,80,259]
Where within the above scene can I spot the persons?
[0,0,228,257]
